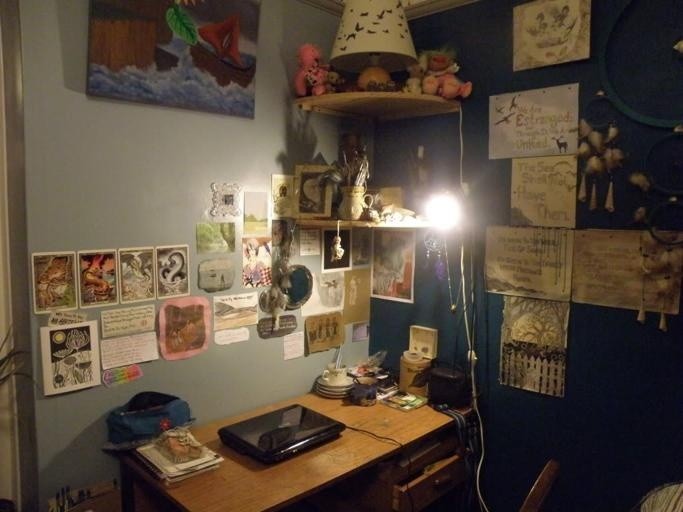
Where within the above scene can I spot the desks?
[121,389,476,512]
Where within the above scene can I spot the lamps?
[328,1,419,90]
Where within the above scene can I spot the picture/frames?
[292,162,334,219]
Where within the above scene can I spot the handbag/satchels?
[107,391,192,443]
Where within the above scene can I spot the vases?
[337,188,374,219]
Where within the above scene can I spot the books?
[377,389,428,413]
[132,428,224,488]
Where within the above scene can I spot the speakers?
[428,367,472,408]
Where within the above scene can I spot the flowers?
[317,142,372,187]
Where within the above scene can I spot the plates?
[313,374,354,400]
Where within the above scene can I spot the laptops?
[217,403,345,464]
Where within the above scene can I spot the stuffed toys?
[295,43,341,97]
[401,49,472,99]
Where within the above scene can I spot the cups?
[321,362,348,382]
